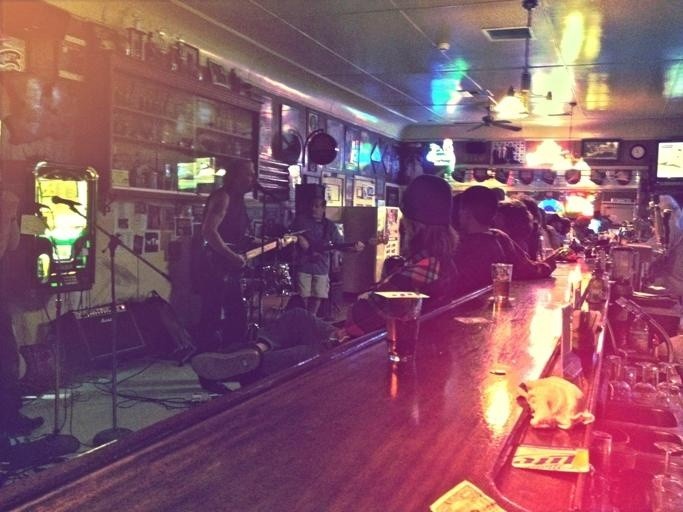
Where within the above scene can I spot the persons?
[192,156,283,394]
[0,191,45,440]
[192,176,457,383]
[282,192,365,318]
[451,182,682,300]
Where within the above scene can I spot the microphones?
[52,196,80,204]
[253,182,273,196]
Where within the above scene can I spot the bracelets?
[9,215,17,223]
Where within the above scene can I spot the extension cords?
[191,394,221,407]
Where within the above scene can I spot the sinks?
[601,399,679,429]
[597,423,683,457]
[617,310,680,354]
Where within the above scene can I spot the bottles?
[588,248,609,305]
[158,164,175,189]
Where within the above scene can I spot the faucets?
[615,296,674,363]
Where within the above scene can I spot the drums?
[245,261,297,312]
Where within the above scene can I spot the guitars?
[205,229,298,284]
[283,230,388,266]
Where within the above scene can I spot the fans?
[454,106,522,133]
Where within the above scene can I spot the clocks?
[630,144,647,160]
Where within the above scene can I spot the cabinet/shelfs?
[101,48,265,203]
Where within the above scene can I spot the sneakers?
[203,381,234,395]
[2,412,44,437]
[191,343,260,381]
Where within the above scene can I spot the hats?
[454,185,500,215]
[400,174,451,226]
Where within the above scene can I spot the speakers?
[295,184,324,223]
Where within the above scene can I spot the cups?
[490,262,514,304]
[384,291,424,363]
[588,352,682,512]
